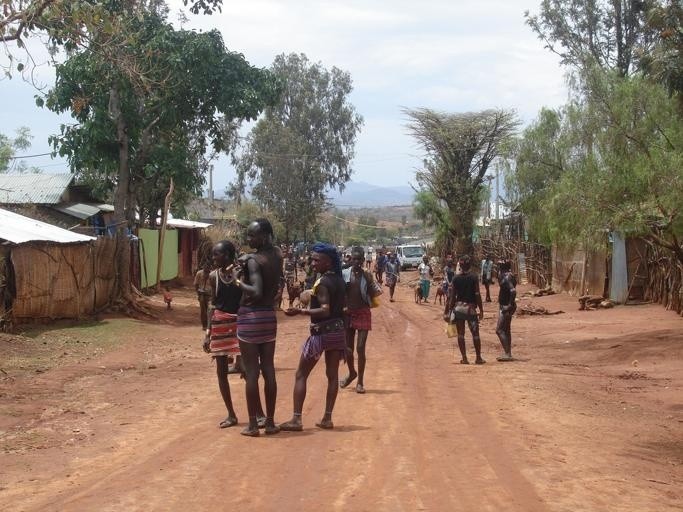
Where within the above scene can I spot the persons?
[164,286,172,310]
[495,261,517,361]
[193,218,493,436]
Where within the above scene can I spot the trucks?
[390,244,425,271]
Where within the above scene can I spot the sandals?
[219,355,515,437]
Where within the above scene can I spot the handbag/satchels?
[369,278,382,308]
[453,301,479,319]
[444,317,458,339]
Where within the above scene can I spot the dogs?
[295,288,313,308]
[415,283,423,304]
[287,280,304,308]
[434,287,446,306]
